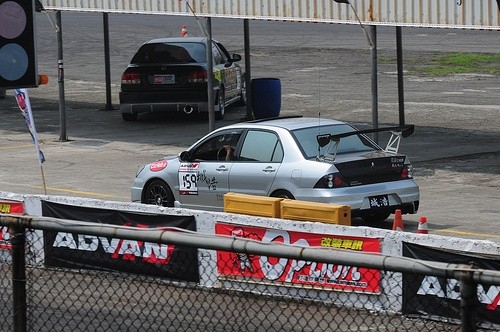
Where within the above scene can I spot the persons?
[224,145,232,161]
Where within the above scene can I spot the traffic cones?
[181,25,188,37]
[391,209,404,231]
[416,216,429,234]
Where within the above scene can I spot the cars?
[131,118,420,226]
[120,37,246,120]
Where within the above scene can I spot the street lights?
[332,0,378,144]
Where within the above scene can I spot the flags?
[14,88,46,166]
